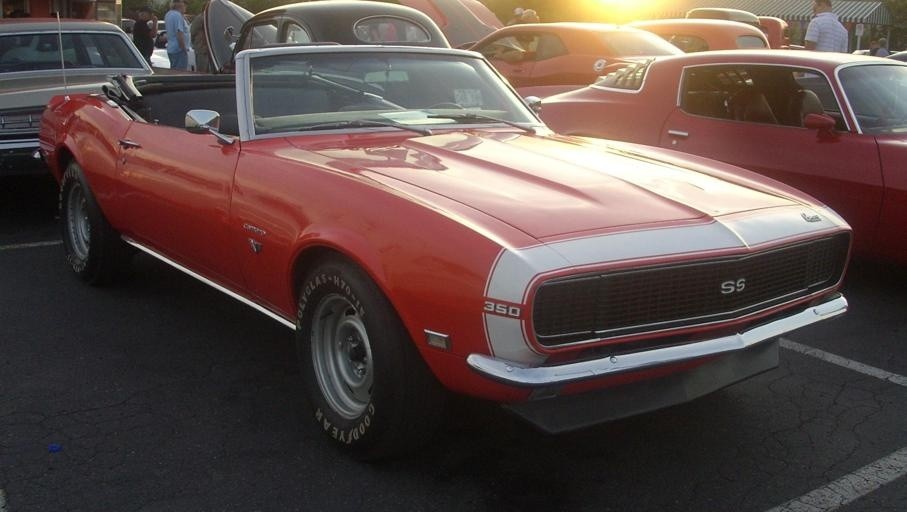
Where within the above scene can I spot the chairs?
[733,88,824,127]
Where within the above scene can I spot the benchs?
[142,86,330,129]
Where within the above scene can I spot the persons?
[506,8,541,48]
[874,37,889,57]
[132,6,158,70]
[802,1,849,55]
[162,0,190,73]
[869,41,879,57]
